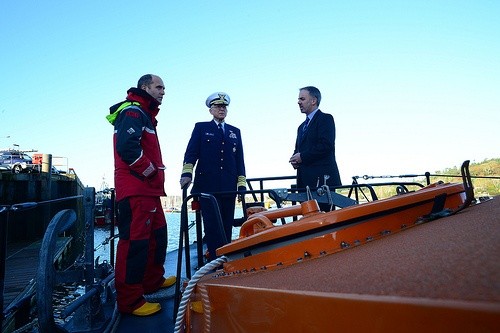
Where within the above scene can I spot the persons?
[179,92,247,272]
[106,73,178,318]
[289,85,343,214]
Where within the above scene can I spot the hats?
[206,92,230,108]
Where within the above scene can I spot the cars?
[0,153,33,174]
[51,165,60,175]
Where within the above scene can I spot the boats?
[95,177,119,228]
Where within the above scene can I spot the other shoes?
[161,275,177,287]
[132,301,161,315]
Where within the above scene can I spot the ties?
[303,117,310,132]
[217,123,224,136]
